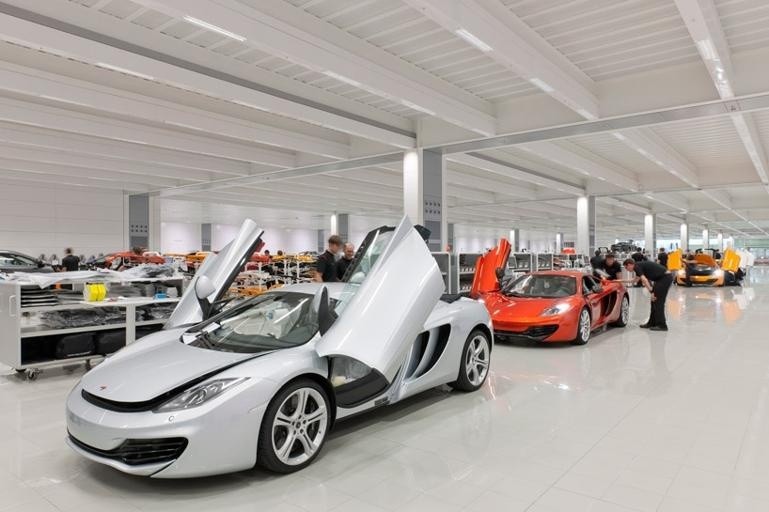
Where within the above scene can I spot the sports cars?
[65,215,495,482]
[470,239,631,346]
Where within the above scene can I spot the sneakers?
[640,323,666,330]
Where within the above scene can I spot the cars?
[0,244,754,291]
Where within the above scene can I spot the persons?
[61,248,80,272]
[590,248,694,331]
[313,235,343,282]
[336,242,362,282]
[716,249,721,259]
[265,250,272,258]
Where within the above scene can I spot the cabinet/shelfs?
[359,251,478,295]
[163,246,318,302]
[503,251,661,288]
